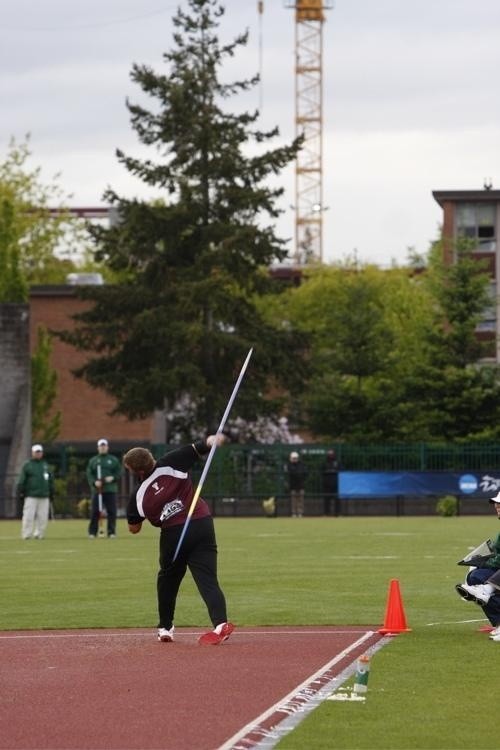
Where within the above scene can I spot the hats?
[489,488,500,503]
[32,444,43,451]
[97,439,108,446]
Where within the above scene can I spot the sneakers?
[480,625,495,631]
[455,583,491,606]
[489,634,500,642]
[198,623,234,645]
[489,626,500,634]
[158,625,174,642]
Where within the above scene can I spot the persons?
[123,433,235,646]
[284,451,307,517]
[455,487,500,643]
[320,450,344,517]
[86,438,122,539]
[17,444,54,540]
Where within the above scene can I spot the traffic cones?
[379,580,412,634]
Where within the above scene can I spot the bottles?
[353,655,371,698]
